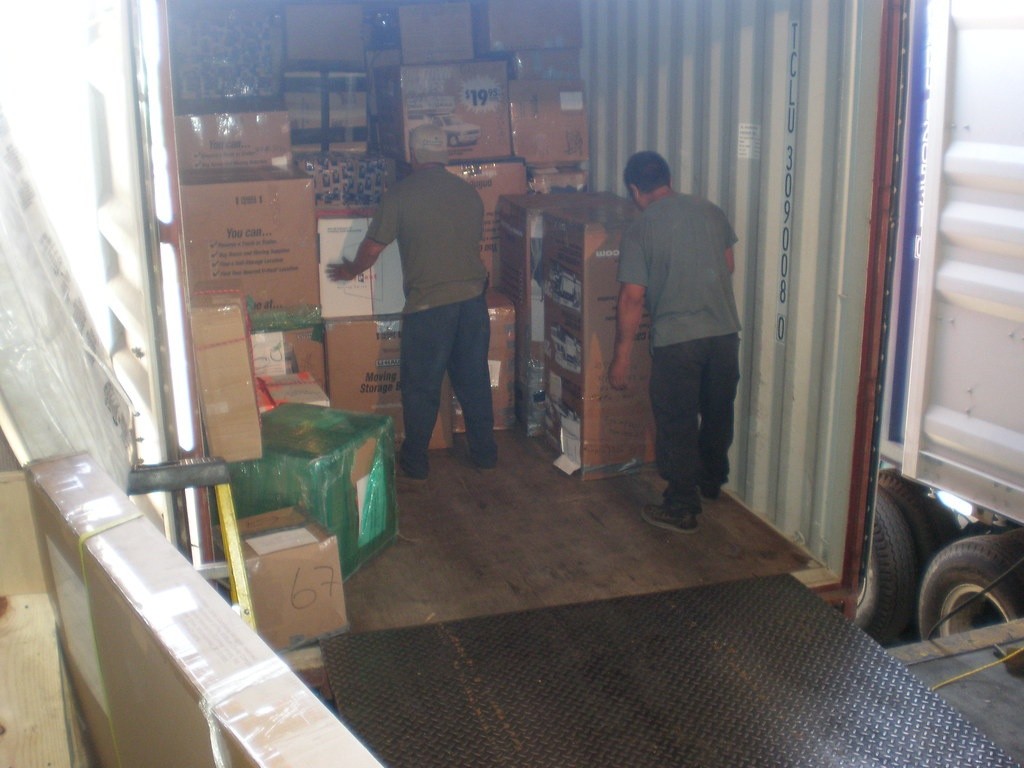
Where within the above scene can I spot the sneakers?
[697,486,721,504]
[642,503,700,534]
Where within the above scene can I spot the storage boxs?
[166,0,661,719]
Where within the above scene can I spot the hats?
[409,124,450,165]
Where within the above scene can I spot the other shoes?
[465,446,496,469]
[393,467,428,484]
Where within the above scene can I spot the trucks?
[3,2,1024,768]
[825,3,1024,649]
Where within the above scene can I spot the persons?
[607,149,744,536]
[324,123,499,479]
